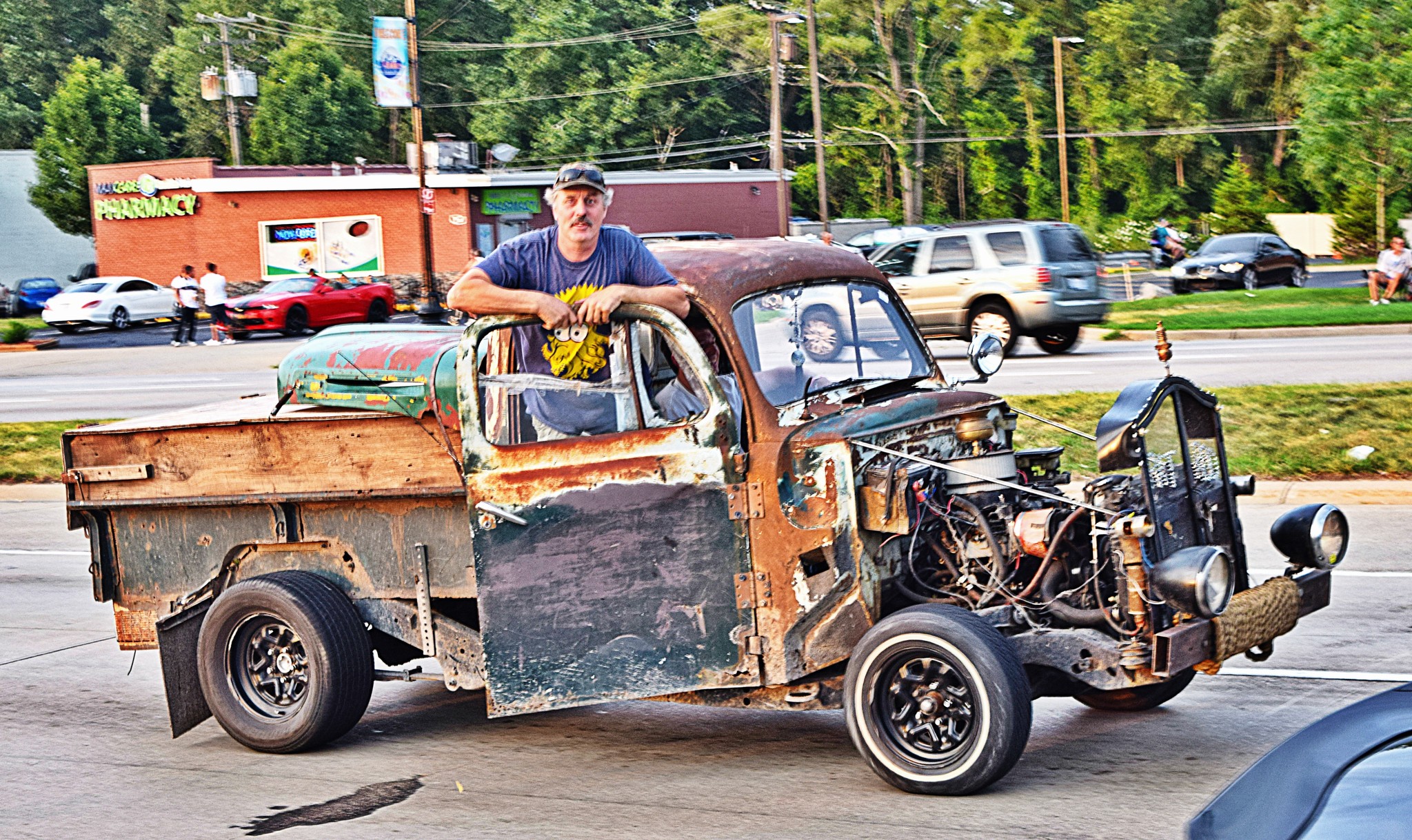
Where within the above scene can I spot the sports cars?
[224,278,396,341]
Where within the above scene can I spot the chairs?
[1360,268,1412,303]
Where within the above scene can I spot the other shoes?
[203,339,221,346]
[170,340,180,347]
[220,338,237,344]
[186,340,198,346]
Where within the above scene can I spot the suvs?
[791,219,1110,365]
[67,263,97,283]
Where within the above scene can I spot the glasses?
[1391,241,1406,245]
[553,169,605,189]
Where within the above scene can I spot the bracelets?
[810,374,826,387]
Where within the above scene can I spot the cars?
[1169,233,1307,295]
[61,239,1349,797]
[7,278,62,318]
[42,276,181,335]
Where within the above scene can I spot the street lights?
[768,12,808,180]
[1053,36,1085,224]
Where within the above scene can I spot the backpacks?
[1149,230,1166,241]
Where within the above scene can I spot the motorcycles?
[1146,232,1190,270]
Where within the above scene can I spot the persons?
[198,262,236,346]
[338,274,351,284]
[170,265,200,348]
[1368,235,1412,306]
[445,160,693,445]
[365,274,376,284]
[308,269,321,278]
[1152,218,1187,262]
[656,315,830,422]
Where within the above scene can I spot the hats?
[552,160,606,194]
